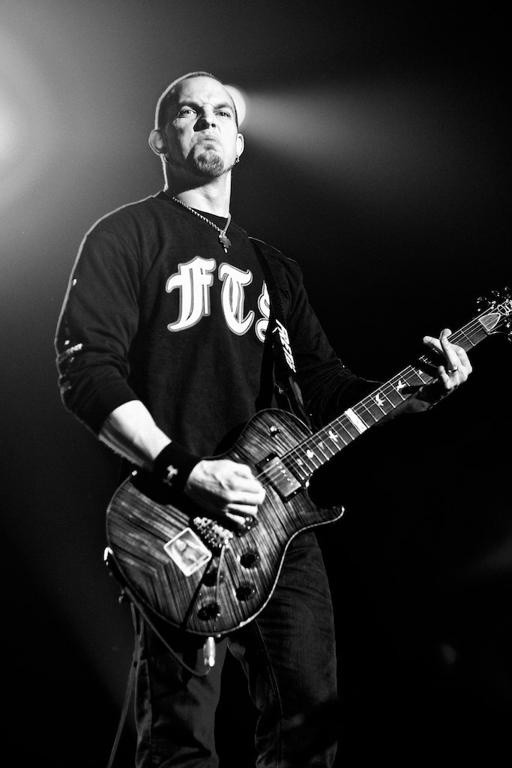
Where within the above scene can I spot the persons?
[57,71,473,768]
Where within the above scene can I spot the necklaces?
[170,195,232,254]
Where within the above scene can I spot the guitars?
[105,286,511,640]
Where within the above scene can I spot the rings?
[448,366,459,374]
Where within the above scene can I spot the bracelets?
[150,440,202,496]
